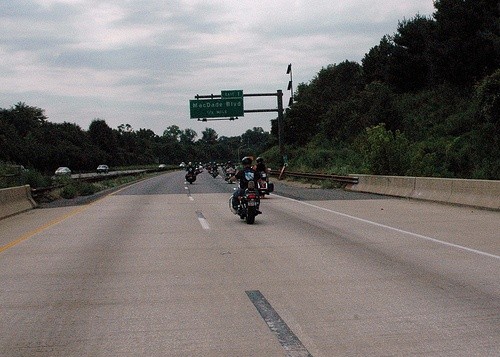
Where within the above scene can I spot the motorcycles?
[187,171,195,185]
[256,171,275,199]
[198,166,237,184]
[226,178,264,224]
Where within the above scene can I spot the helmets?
[241,157,253,165]
[256,157,263,163]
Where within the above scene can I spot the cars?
[159,163,166,169]
[54,167,73,177]
[97,165,110,173]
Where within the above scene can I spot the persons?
[185,162,200,173]
[228,157,262,215]
[255,157,268,181]
[199,162,204,169]
[207,160,235,176]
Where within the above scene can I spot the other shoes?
[233,206,238,210]
